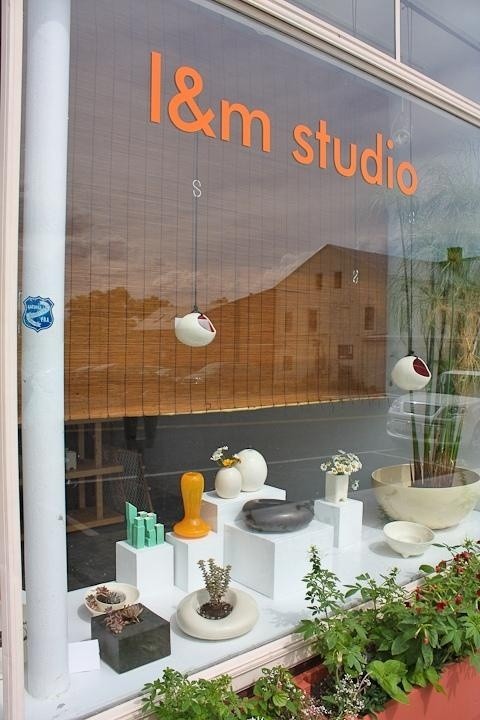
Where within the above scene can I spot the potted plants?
[364,127,480,529]
[91,602,171,674]
[176,559,259,641]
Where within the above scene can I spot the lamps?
[390,94,411,146]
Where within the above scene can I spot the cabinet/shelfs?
[64,417,130,538]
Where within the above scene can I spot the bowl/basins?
[382,521,437,558]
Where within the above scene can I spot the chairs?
[113,446,158,514]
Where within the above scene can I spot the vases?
[172,471,212,539]
[234,448,268,492]
[214,467,242,498]
[325,472,349,502]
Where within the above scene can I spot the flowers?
[319,449,362,491]
[210,445,241,468]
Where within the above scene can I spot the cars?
[386,367,477,452]
[63,355,236,398]
